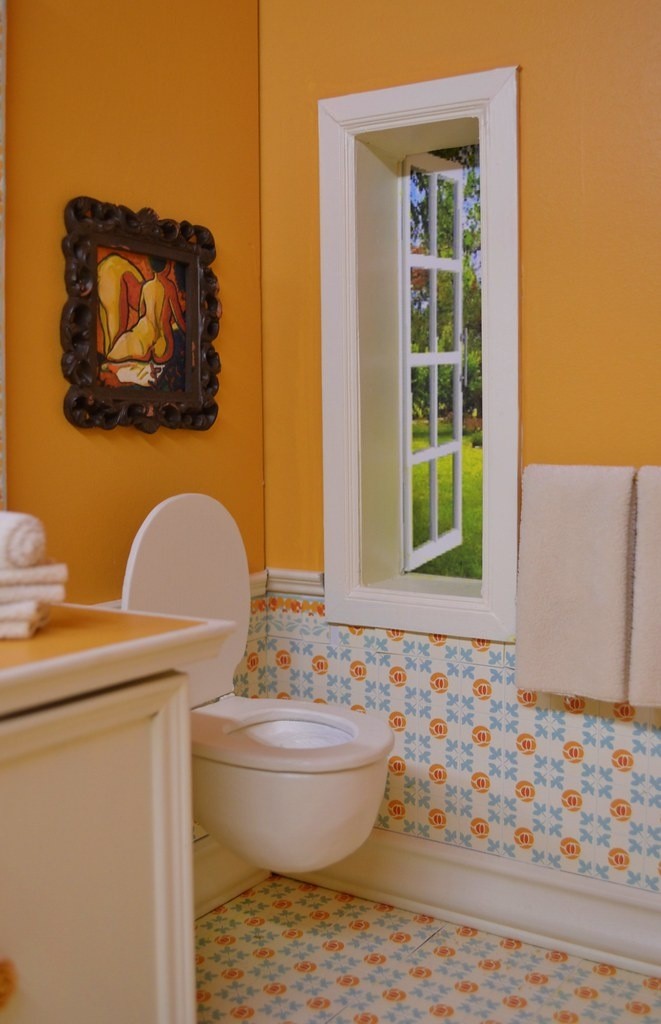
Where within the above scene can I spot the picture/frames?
[60,197,221,432]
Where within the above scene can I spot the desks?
[0,606,238,1024]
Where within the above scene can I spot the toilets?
[121,493,396,874]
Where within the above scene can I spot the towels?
[514,462,661,709]
[0,510,71,640]
